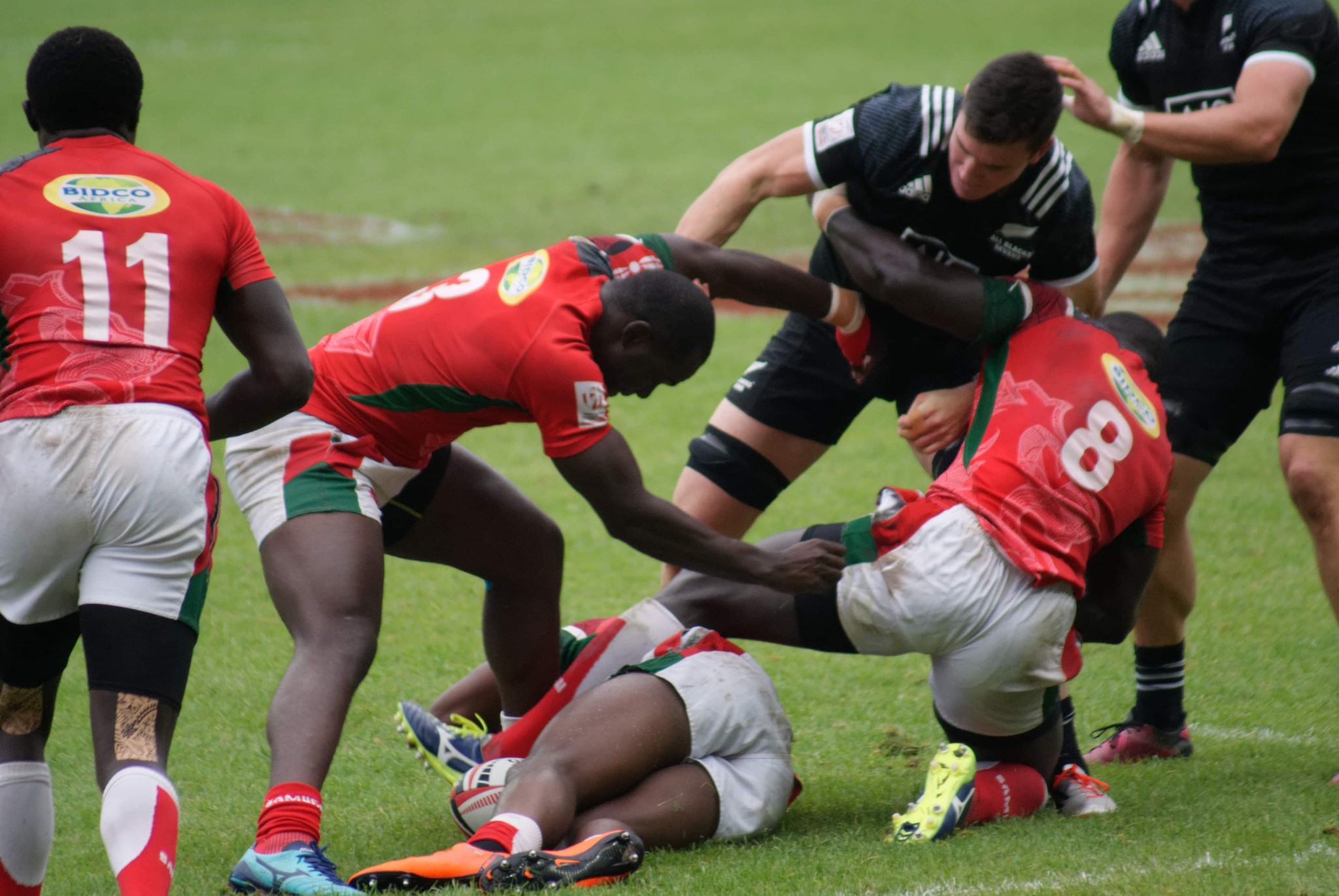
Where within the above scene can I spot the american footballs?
[448,758,527,840]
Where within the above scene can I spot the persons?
[653,184,1174,847]
[345,616,804,896]
[1042,0,1339,767]
[0,28,317,896]
[659,52,1114,819]
[223,231,869,896]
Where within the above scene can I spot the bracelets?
[811,188,851,237]
[821,282,865,333]
[1108,102,1143,147]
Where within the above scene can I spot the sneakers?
[347,843,510,893]
[395,701,492,786]
[1049,763,1117,818]
[883,743,977,844]
[1082,706,1193,765]
[228,840,366,896]
[478,830,644,896]
